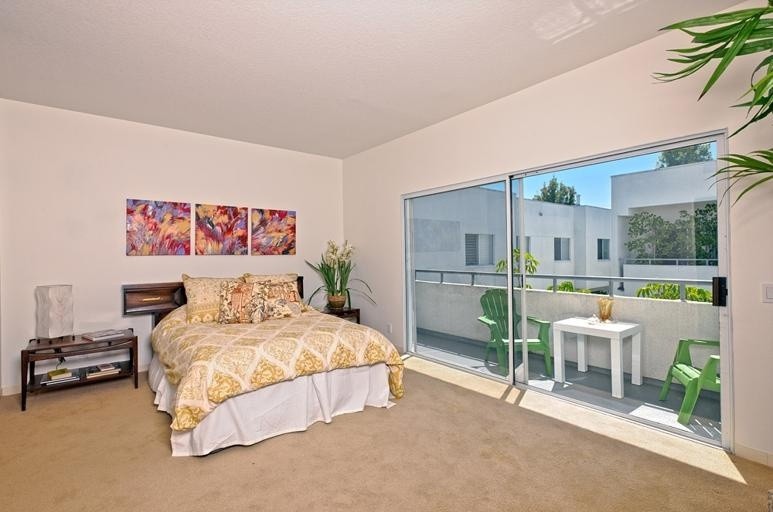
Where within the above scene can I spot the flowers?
[305,241,376,310]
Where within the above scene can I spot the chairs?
[477,288,553,378]
[659,339,720,426]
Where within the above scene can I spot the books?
[82,329,125,341]
[86,360,121,379]
[39,368,80,385]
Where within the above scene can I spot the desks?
[21,328,138,411]
[552,316,643,399]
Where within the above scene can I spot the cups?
[598,297,615,321]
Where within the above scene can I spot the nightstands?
[319,307,360,324]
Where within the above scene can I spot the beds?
[122,276,404,457]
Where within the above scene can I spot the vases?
[327,296,345,309]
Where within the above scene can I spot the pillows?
[269,281,307,319]
[220,280,271,324]
[244,272,298,284]
[182,272,237,325]
